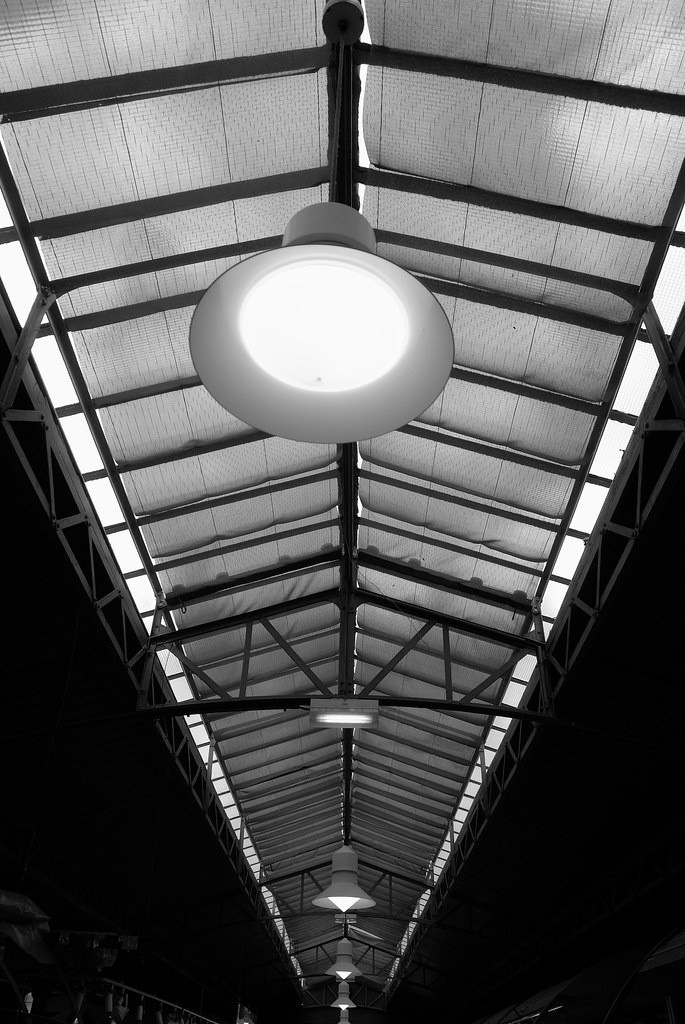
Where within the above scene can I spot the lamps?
[330,981,357,1011]
[308,698,380,729]
[338,1009,351,1024]
[187,202,454,445]
[312,845,376,913]
[324,938,363,980]
